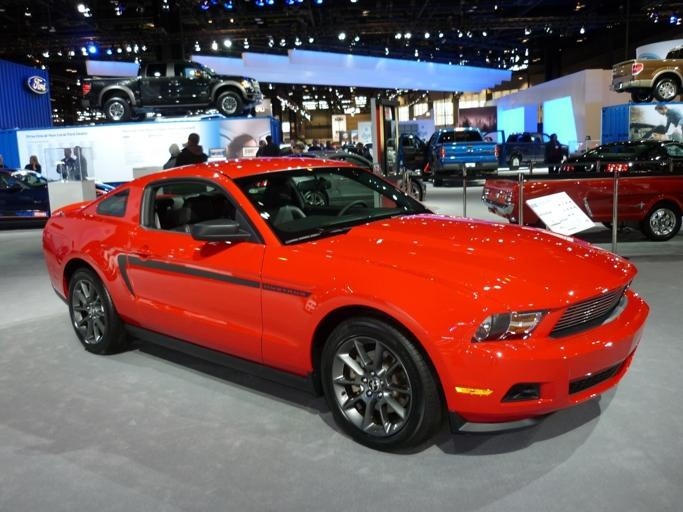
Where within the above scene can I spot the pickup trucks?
[80,57,264,124]
[481,137,683,242]
[611,45,683,103]
[396,128,570,188]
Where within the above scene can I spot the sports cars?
[0,166,117,229]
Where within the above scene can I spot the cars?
[42,150,650,454]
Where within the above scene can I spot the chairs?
[185,195,236,227]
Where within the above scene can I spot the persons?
[22,154,42,176]
[258,118,500,173]
[227,134,256,163]
[72,144,87,181]
[172,132,210,168]
[55,149,75,178]
[544,134,564,174]
[654,104,682,142]
